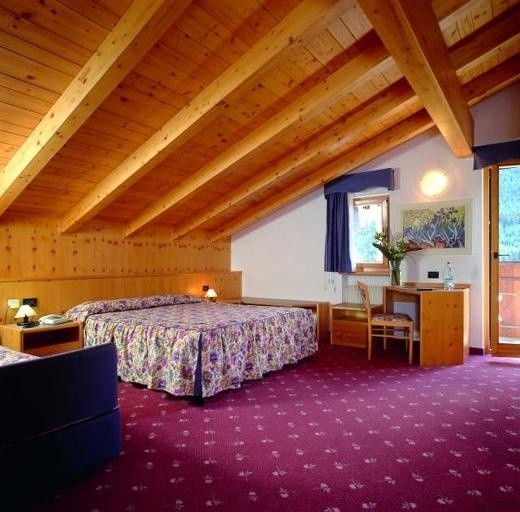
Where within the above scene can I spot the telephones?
[38,314,69,325]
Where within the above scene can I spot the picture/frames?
[400,198,472,255]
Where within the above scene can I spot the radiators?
[348,286,383,304]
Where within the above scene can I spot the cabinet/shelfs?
[383,280,469,365]
[329,302,383,347]
[235,297,329,341]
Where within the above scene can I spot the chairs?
[358,280,413,365]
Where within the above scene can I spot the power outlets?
[9,299,20,308]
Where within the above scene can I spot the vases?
[389,259,402,285]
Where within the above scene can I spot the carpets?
[57,343,520,512]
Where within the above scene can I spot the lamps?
[14,305,38,325]
[205,289,217,302]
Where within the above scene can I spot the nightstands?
[0,324,84,356]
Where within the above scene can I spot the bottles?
[444,262,455,291]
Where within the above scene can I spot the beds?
[0,343,120,512]
[66,302,318,402]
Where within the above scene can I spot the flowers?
[372,232,424,285]
